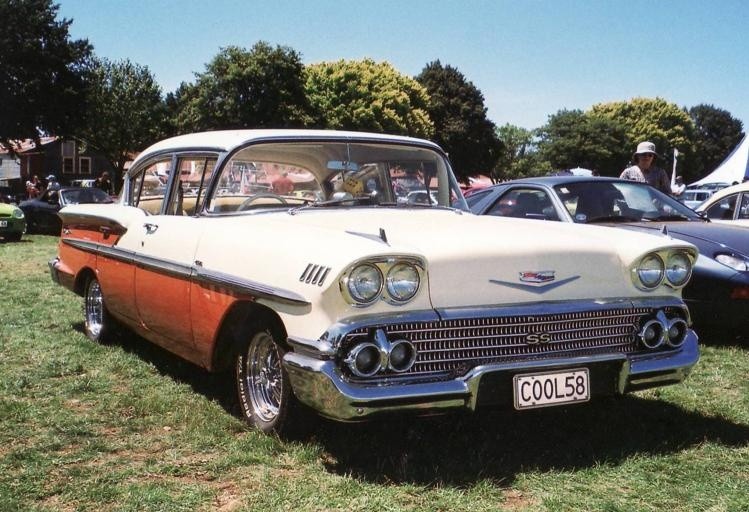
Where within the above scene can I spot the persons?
[671,175,687,214]
[727,180,743,215]
[416,168,426,189]
[616,140,672,220]
[26,175,60,202]
[94,171,112,202]
[742,176,749,197]
[272,171,294,195]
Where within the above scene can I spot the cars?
[668,181,749,214]
[18,186,115,236]
[47,130,703,438]
[0,193,26,240]
[694,183,749,229]
[449,175,749,326]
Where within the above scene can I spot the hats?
[46,175,56,180]
[635,141,658,157]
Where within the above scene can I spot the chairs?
[509,192,547,218]
[574,192,606,221]
